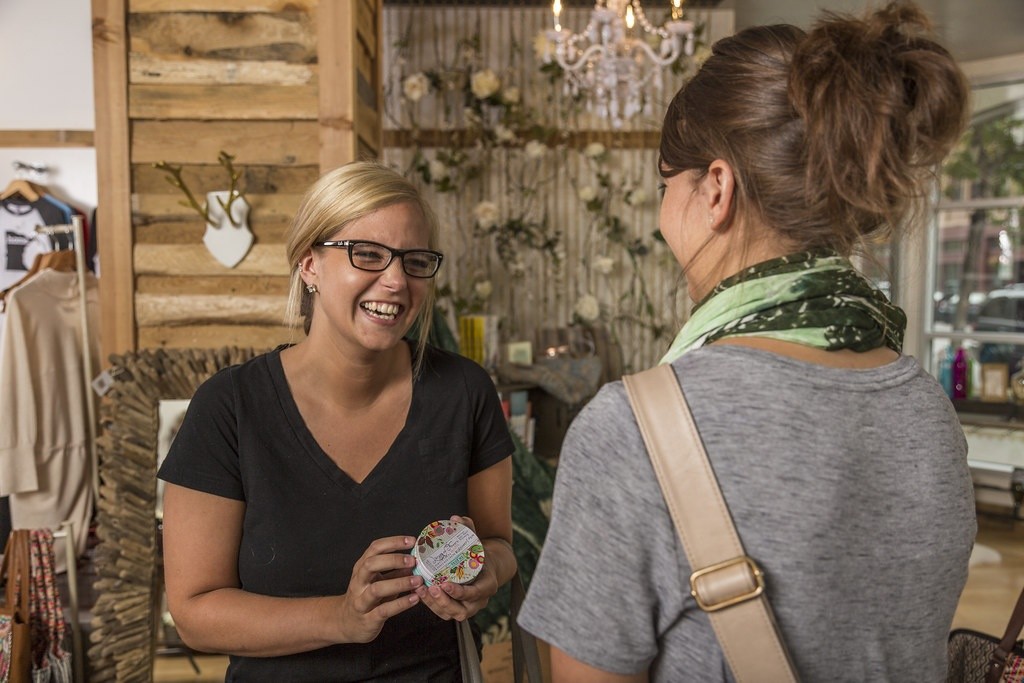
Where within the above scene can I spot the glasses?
[313,239,444,278]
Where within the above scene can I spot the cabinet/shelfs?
[9,214,103,683]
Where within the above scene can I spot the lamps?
[552,0,696,128]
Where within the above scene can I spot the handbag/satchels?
[0,529,33,683]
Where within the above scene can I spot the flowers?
[381,14,683,370]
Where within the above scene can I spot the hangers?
[1,162,96,314]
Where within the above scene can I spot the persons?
[517,0,976,682]
[156,161,517,682]
